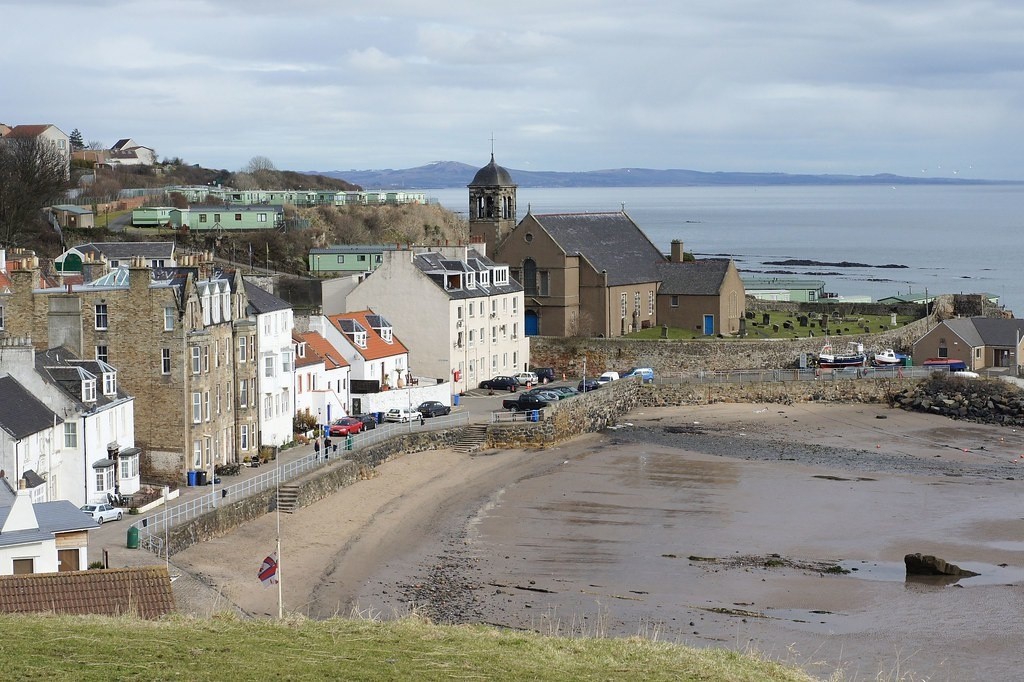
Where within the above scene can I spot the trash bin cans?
[197,470,207,486]
[324,425,330,438]
[454,394,460,406]
[532,409,541,423]
[188,470,198,486]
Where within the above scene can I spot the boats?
[874,343,901,365]
[817,324,867,368]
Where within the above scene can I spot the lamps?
[458,321,462,327]
[491,313,495,318]
[500,325,505,330]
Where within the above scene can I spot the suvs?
[620,367,654,383]
[513,372,539,387]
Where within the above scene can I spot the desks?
[121,496,133,507]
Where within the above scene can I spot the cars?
[530,386,580,400]
[350,415,377,432]
[577,380,599,392]
[79,504,123,524]
[480,376,520,390]
[418,401,451,418]
[385,408,422,423]
[330,417,362,436]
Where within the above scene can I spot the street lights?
[317,408,322,464]
[407,366,411,431]
[204,435,214,507]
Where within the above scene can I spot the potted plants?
[395,369,404,388]
[384,374,389,391]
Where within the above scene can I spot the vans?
[530,368,554,384]
[597,372,620,385]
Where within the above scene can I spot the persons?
[324,436,332,459]
[315,438,319,459]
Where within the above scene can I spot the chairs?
[107,493,128,506]
[405,374,418,385]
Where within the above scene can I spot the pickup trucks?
[503,394,553,412]
